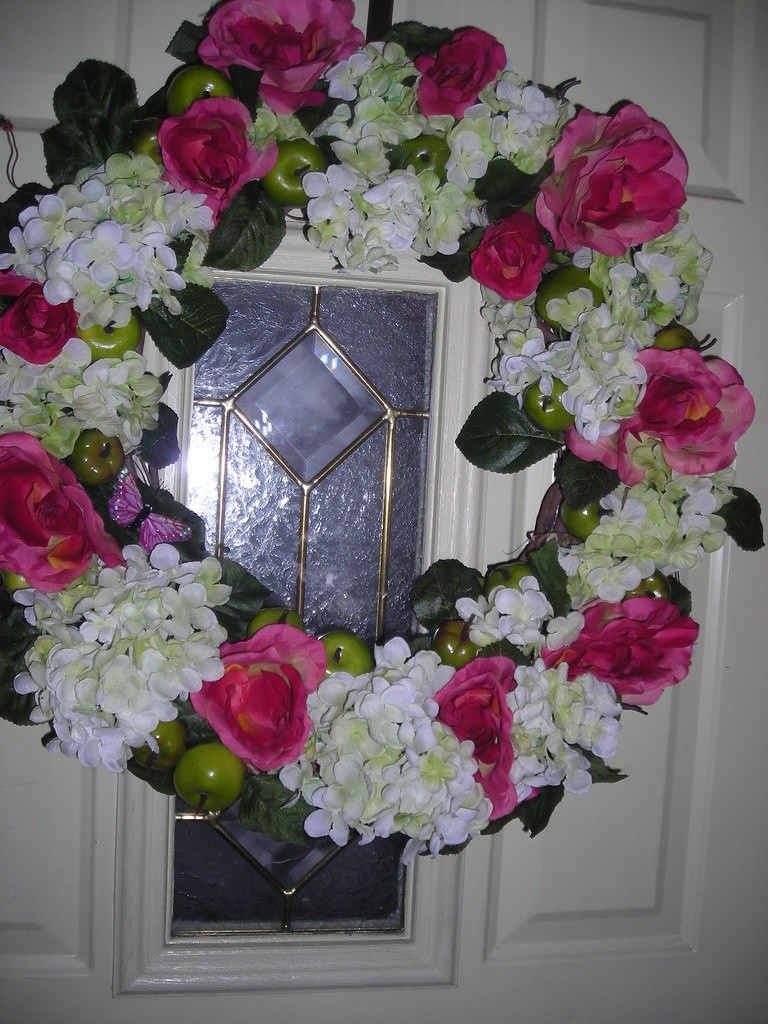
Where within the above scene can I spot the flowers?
[1,0,765,864]
